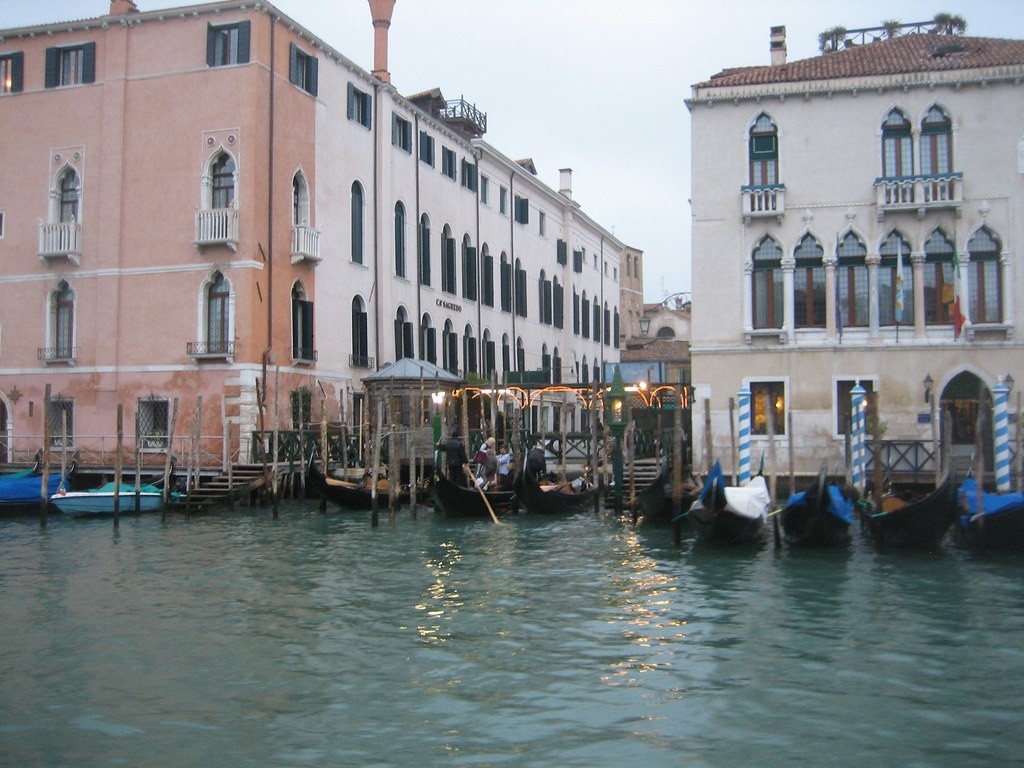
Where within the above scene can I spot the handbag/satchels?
[474,452,488,464]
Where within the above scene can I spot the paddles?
[468,466,501,524]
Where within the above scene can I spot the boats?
[430,451,607,518]
[779,456,860,545]
[308,456,436,511]
[51,481,179,516]
[0,446,80,510]
[859,452,961,554]
[687,452,771,543]
[954,450,1024,544]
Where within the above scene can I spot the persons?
[525,445,546,479]
[441,431,514,492]
[669,461,704,495]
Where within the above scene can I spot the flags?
[895,246,905,322]
[951,237,967,337]
